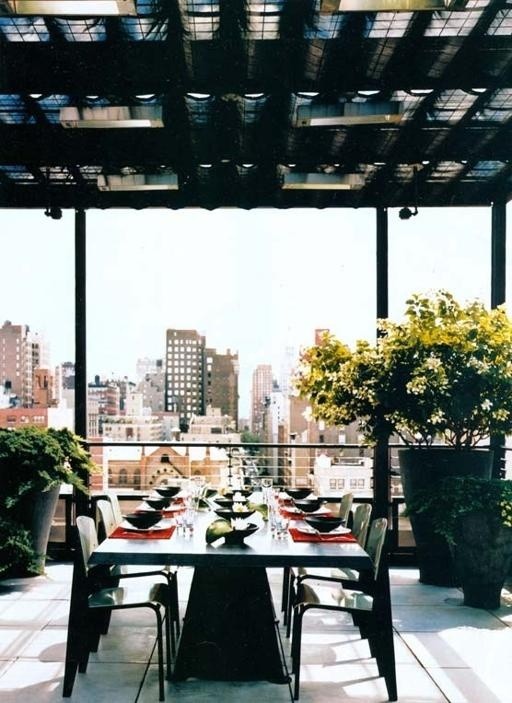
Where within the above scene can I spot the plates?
[121,487,352,533]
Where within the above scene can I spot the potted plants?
[400,475,512,609]
[0,425,113,578]
[291,288,512,587]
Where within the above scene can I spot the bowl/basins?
[122,486,346,544]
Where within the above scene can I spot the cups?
[173,475,291,539]
